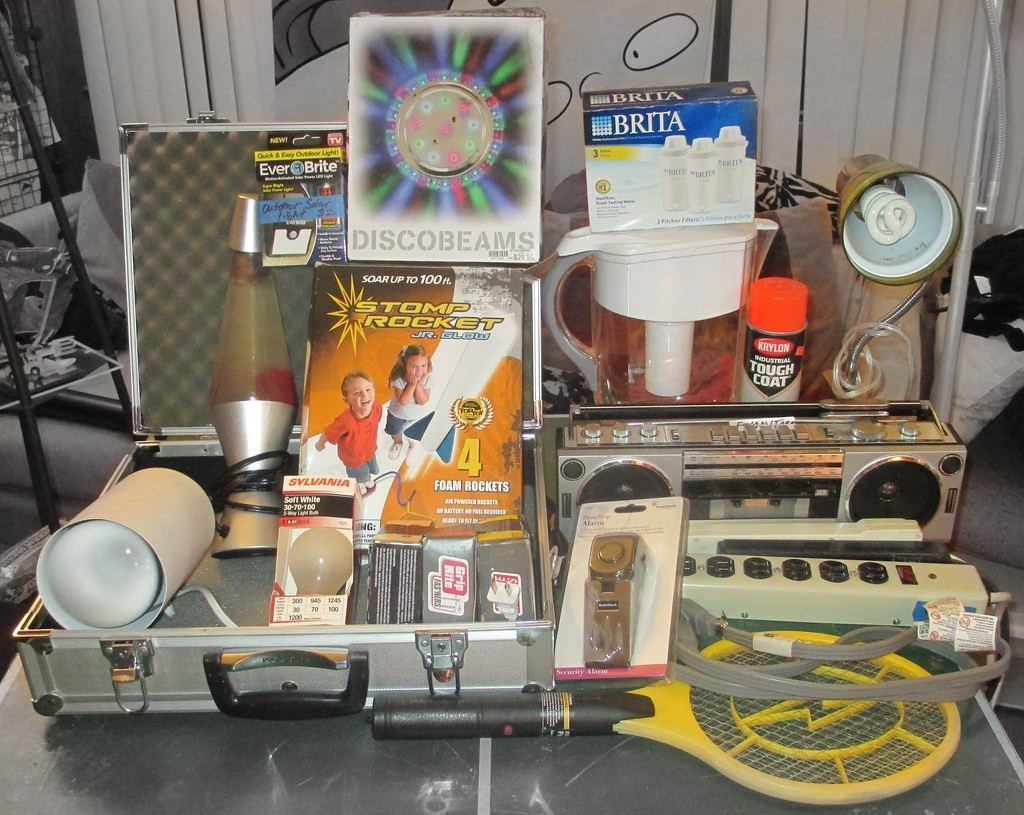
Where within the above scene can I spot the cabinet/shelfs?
[0,2,133,535]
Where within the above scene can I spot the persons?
[384,345,434,460]
[315,370,382,496]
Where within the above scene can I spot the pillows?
[749,163,1024,444]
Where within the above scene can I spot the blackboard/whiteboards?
[120,122,351,438]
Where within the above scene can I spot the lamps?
[36,464,241,632]
[822,155,963,406]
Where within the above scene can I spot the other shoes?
[358,477,375,495]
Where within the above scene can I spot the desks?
[0,654,1024,815]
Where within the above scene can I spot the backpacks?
[941,230,1024,354]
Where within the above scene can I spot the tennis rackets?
[370,627,961,806]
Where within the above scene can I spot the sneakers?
[406,447,420,465]
[388,442,403,460]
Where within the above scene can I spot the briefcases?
[13,113,556,717]
